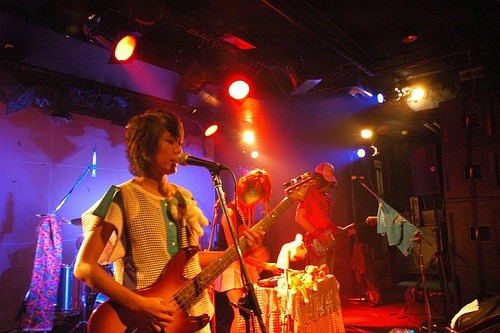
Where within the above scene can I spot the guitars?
[87,173,316,332]
[305,216,378,255]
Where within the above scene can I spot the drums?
[58,265,83,311]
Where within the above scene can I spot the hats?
[315,162,337,183]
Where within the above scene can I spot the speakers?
[454,294,500,333]
[438,91,500,310]
[350,160,384,242]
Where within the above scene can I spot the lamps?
[81,12,142,66]
[282,55,323,96]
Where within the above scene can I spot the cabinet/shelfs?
[393,226,450,274]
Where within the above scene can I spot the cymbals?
[71,218,82,226]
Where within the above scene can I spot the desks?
[236,274,346,333]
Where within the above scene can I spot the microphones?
[351,175,368,181]
[91,152,97,178]
[177,152,229,171]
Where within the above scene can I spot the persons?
[294,162,344,274]
[73,108,266,333]
[215,168,284,333]
